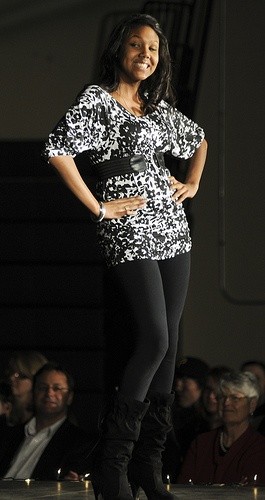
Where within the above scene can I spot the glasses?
[215,394,250,402]
[12,368,32,380]
[34,383,69,393]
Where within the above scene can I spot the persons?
[170,357,265,486]
[0,344,63,484]
[0,361,103,500]
[184,373,264,486]
[43,13,207,500]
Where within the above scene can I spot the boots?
[92,392,151,500]
[127,391,181,500]
